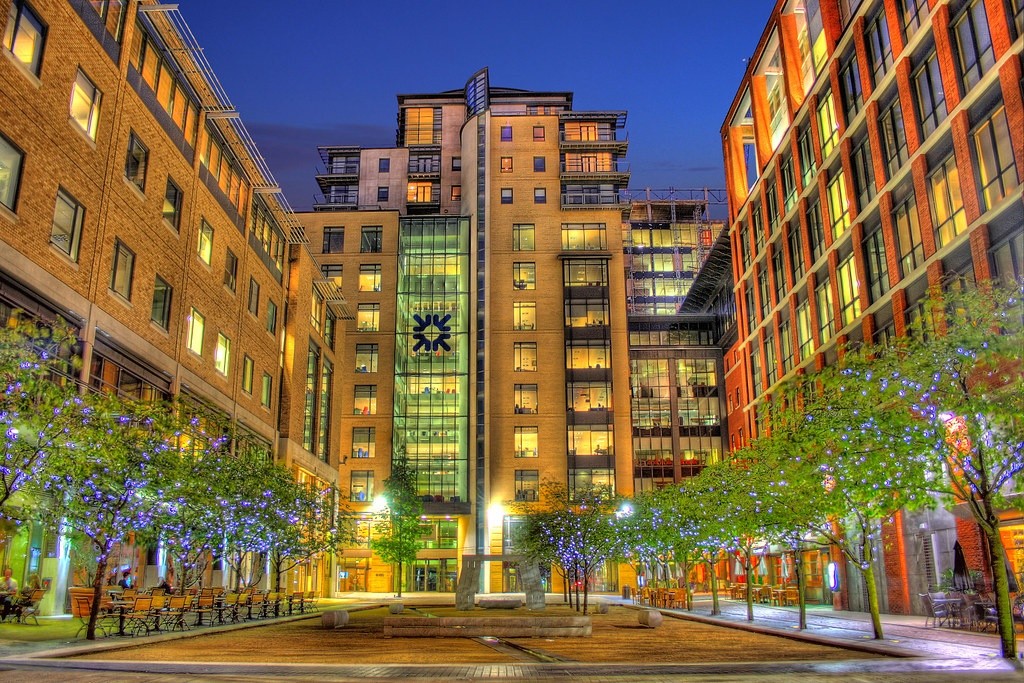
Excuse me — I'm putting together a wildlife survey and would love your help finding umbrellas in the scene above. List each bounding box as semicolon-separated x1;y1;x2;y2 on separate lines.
643;559;684;586
950;540;975;594
992;538;1019;594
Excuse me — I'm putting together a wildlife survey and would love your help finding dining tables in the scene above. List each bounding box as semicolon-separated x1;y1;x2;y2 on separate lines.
190;599;209;626
0;591;17;625
150;600;169;632
214;598;228;623
753;588;773;604
773;589;796;607
934;599;965;629
106;601;138;637
975;602;993;632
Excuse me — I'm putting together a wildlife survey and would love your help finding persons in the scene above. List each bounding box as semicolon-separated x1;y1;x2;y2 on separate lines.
119;572;134;590
158;577;171;595
16;574;40;623
0;568;18;623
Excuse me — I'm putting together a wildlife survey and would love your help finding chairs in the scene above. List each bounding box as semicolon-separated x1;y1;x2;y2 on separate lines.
67;584;321;639
917;591;1004;634
631;587;692;610
723;583;801;607
10;588;47;625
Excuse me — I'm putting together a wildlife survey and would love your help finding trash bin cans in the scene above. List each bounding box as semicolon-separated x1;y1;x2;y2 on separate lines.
622;584;631;600
